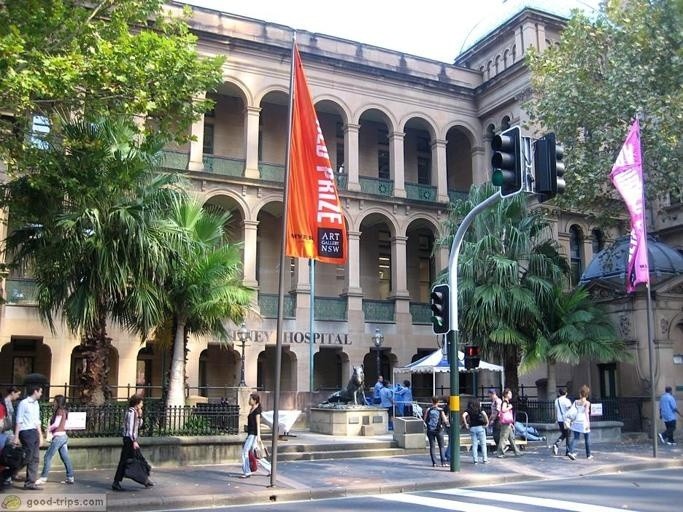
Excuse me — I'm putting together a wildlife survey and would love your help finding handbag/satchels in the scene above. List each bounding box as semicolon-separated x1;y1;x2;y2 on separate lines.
0;416;11;433
497;410;513;423
123;448;146;485
254;440;269;459
477;413;484;423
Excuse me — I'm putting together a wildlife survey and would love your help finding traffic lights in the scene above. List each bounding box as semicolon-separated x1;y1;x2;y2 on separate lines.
534;132;566;203
430;284;449;334
491;125;521;198
464;345;481;370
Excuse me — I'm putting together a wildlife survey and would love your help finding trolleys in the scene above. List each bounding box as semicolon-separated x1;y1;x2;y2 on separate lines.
478;410;528;450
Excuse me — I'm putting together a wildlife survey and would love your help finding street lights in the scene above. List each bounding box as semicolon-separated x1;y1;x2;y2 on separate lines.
372;327;385;376
236;323;251;387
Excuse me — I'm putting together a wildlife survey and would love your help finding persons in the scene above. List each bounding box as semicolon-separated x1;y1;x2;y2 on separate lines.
111;395;157;490
0;381;74;489
564;385;593;460
239;393;271;478
553;387;577;456
361;375;546;467
658;386;682;445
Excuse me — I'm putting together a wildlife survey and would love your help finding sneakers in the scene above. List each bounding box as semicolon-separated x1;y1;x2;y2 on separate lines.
666;440;677;444
442;463;450;467
433;463;442;467
587;454;593;459
658;433;665;444
566;452;575;459
553;444;558;455
59;480;73;484
35;480;46;485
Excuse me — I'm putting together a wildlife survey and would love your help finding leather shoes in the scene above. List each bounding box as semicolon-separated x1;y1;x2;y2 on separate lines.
23;484;42;490
144;481;155;487
111;482;121;490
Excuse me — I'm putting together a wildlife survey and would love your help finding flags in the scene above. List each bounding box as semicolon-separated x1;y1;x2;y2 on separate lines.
608;120;649;294
283;45;348;265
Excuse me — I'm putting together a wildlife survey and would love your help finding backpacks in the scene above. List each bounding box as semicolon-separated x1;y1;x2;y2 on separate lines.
425;407;441;432
